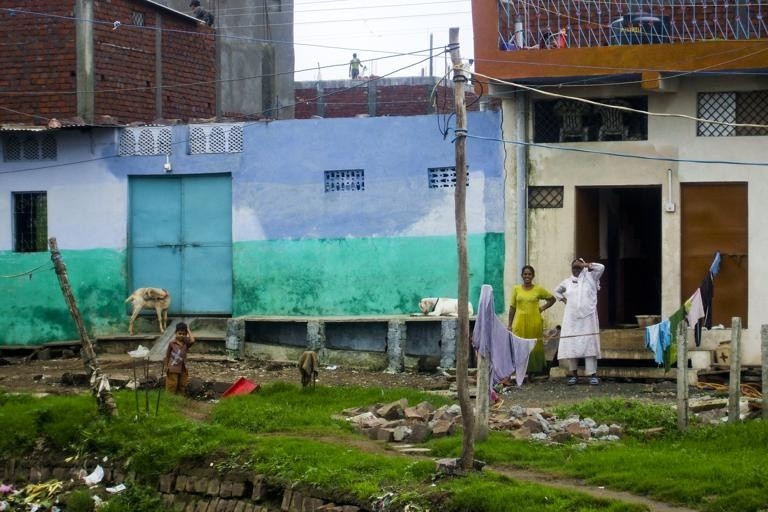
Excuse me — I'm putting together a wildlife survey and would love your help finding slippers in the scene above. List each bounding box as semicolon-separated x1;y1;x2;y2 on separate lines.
498;379;515;387
492;399;504;410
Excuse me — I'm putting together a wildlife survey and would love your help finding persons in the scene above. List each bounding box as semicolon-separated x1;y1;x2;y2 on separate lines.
348;52;363;79
189;1;216;28
550;257;607;387
505;262;554;385
161;320;196;399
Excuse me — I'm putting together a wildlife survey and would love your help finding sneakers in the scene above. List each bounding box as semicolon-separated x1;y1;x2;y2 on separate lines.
589;376;600;385
568;375;578;385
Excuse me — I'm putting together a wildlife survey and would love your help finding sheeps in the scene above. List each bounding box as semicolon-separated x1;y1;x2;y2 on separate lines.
412;298;474;320
124;287;172;335
